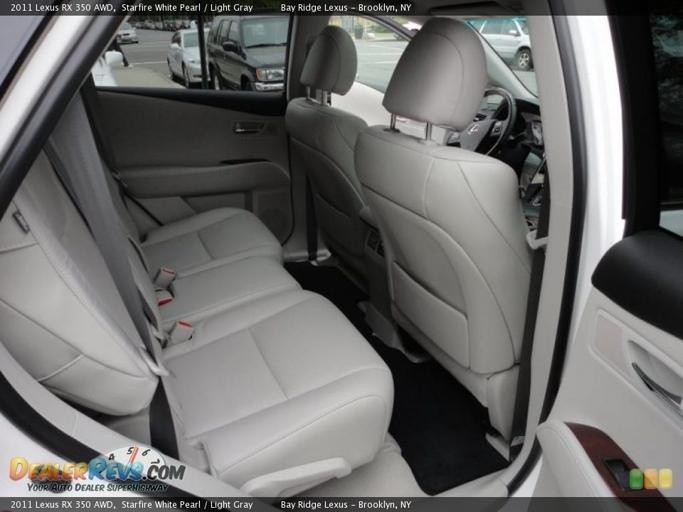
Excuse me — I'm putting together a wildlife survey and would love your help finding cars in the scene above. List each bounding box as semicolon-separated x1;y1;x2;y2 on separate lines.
166;28;212;87
0;1;680;510
131;19;208;31
395;21;422;39
116;23;140;44
463;18;534;72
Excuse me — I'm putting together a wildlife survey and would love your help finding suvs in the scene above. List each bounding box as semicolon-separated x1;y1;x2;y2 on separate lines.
207;15;292;92
